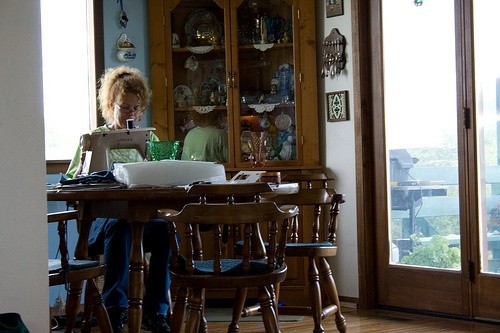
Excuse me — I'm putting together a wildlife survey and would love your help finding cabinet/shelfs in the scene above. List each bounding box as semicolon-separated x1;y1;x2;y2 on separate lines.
147;0;322;166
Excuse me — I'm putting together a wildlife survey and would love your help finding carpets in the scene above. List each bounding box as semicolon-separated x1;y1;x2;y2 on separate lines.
185;306;304;322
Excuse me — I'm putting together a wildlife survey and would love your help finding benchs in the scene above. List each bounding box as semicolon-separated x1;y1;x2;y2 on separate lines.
392;166;500;260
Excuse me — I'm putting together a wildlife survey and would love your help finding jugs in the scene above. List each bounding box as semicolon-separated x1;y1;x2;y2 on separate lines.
171;33;179;45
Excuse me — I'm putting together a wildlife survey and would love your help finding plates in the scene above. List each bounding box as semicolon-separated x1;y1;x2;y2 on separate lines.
274;114;292;129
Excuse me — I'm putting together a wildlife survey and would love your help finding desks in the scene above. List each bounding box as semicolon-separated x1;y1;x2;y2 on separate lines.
47;181;298;333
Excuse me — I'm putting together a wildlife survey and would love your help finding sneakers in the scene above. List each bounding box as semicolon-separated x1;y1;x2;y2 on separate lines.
104;301;128;333
140;315;171;333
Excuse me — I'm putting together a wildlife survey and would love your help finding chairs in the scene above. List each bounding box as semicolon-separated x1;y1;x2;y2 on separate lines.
47;171;347;333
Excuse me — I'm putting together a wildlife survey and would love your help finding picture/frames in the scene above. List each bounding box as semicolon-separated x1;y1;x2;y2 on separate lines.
325;90;350;122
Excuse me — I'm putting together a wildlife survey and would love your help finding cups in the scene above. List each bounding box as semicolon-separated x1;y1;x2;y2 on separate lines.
145;140;179;161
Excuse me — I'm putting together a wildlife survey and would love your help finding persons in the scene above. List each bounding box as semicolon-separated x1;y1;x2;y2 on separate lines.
180;79;230;163
64;66;181;333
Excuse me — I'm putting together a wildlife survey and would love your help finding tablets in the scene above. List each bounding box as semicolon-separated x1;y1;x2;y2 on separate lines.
83;130;150;179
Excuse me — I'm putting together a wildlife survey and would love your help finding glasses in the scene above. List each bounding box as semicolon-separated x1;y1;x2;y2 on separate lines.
115;103;144;117
